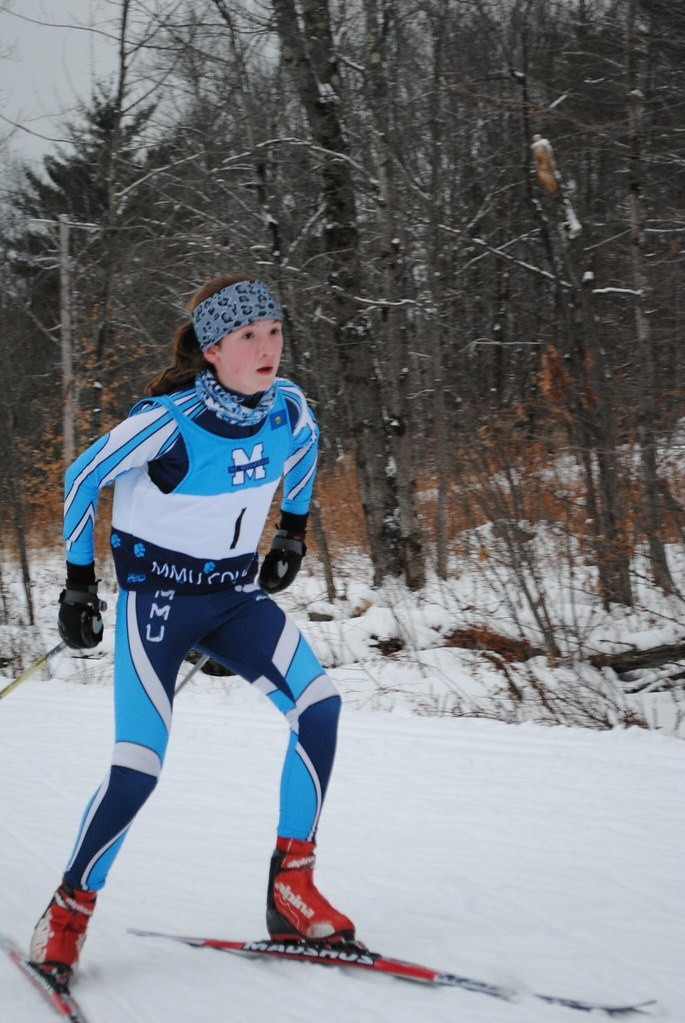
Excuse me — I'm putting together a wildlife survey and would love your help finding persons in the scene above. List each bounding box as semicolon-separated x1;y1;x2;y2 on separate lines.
27;269;359;993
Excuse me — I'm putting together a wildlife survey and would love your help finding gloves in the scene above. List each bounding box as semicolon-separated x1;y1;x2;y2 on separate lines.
57;578;107;650
256;524;308;594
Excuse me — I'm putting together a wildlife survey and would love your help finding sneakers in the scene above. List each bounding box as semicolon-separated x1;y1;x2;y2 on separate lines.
265;835;357;944
30;882;96;984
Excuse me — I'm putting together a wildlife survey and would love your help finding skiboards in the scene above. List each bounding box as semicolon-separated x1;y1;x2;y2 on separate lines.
0;927;660;1023
